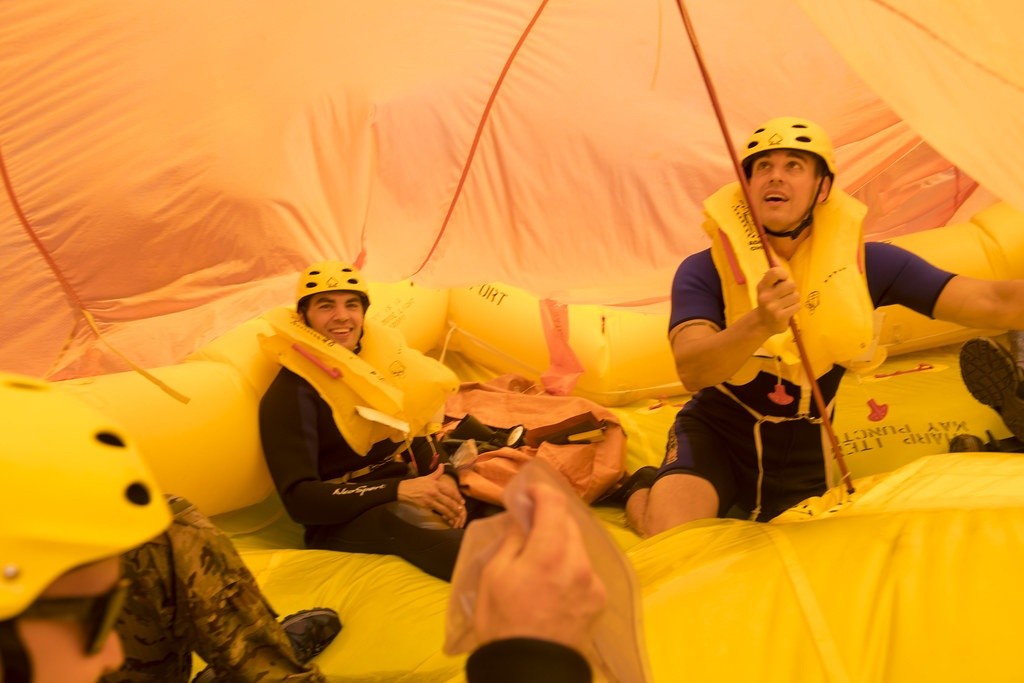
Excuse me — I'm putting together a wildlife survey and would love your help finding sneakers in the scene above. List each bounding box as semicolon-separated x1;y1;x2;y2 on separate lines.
192;607;343;683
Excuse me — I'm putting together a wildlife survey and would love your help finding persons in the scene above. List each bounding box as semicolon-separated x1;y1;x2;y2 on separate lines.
947;336;1023;455
259;260;497;587
598;116;1024;540
0;372;608;683
116;492;342;682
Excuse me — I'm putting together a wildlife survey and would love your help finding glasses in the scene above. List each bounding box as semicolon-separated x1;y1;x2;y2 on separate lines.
21;579;132;654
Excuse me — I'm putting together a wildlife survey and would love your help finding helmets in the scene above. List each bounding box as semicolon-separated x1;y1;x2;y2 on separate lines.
0;371;173;622
739;117;837;176
295;261;370;314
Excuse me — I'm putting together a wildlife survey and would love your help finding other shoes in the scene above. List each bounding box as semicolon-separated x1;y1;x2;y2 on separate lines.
958;334;1024;443
949;434;983;453
